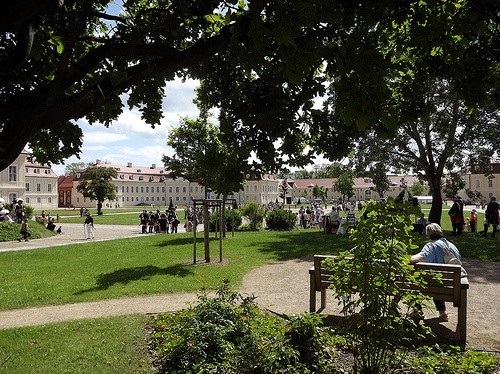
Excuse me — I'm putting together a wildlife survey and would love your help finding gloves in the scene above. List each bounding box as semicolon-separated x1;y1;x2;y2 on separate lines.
89;222;92;224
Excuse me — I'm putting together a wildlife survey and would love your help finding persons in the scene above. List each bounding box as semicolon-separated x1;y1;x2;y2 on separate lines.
385;195;388;204
444;196;500;240
110;202;120;208
409;223;462;322
360;296;402;316
411;213;431;235
139;200;363;235
0;198;95;242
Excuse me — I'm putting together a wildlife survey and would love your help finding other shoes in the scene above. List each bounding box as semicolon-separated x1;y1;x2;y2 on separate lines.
25;240;28;242
409;311;424;319
19;239;21;242
439;312;449;322
87;238;91;239
92;237;94;239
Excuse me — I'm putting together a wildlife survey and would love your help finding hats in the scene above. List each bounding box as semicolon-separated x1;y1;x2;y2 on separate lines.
157;210;160;213
452;196;462;200
149;211;153;213
166;210;169;212
171;208;175;210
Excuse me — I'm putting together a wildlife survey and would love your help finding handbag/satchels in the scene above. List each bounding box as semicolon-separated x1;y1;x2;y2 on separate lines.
451;216;460;223
20;231;25;235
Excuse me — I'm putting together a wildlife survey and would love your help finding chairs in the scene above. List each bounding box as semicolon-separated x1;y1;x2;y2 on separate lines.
157;219;167;234
347;214;356;237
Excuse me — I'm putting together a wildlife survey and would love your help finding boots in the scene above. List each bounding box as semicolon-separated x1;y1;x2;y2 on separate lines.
167;227;170;234
149;228;153;233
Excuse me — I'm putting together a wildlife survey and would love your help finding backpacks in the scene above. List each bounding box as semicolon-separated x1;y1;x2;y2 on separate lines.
434;241;467;277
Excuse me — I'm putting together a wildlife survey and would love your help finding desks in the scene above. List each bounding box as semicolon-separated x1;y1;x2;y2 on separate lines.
411;221;431;235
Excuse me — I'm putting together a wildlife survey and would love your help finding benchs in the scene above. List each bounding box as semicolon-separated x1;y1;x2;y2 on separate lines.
308;254;469;352
321;215;340;233
